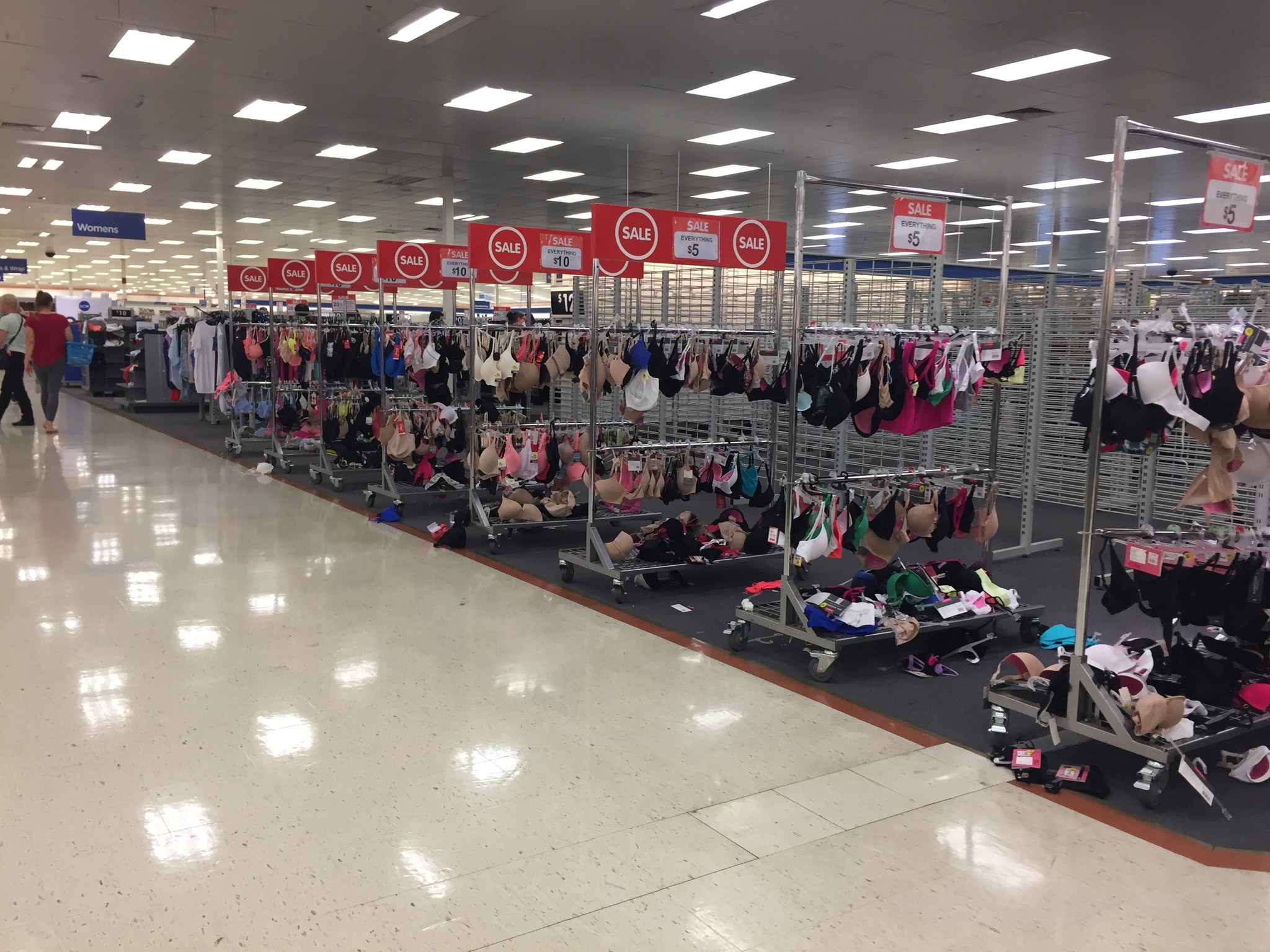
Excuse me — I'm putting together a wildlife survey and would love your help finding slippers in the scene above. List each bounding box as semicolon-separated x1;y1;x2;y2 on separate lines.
42;422;59;435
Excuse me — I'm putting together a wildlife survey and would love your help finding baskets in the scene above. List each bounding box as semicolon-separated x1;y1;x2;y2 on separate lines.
65;335;97;368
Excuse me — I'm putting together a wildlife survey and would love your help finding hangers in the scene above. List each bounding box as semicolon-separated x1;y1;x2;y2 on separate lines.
251;315;1267;568
161;306;249;328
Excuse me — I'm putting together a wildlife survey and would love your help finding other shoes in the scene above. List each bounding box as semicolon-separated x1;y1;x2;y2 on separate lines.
35;378;42;394
9;393;19;404
12;419;35;427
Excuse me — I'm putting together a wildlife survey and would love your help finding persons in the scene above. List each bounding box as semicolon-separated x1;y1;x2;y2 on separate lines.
23;290;73;434
0;293;35;426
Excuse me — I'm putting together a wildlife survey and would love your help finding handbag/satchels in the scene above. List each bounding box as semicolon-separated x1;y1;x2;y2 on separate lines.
0;347;9;370
